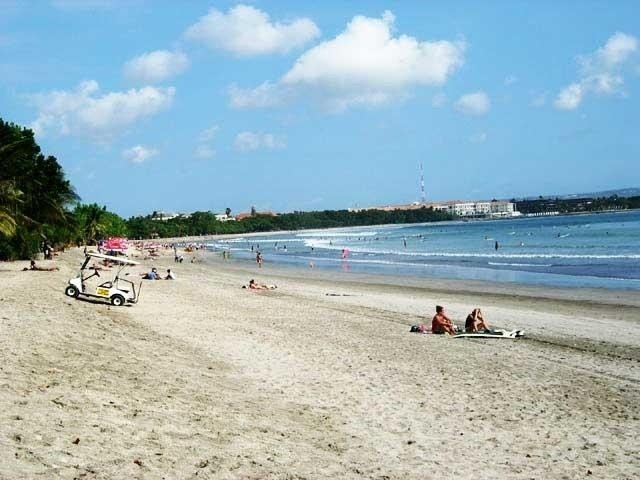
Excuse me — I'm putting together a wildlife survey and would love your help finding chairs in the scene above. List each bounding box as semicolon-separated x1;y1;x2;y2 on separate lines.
100;277;118;288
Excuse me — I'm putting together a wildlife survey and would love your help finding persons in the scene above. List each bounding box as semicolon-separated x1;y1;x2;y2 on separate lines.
96;238;287;290
29;261;60;272
432;306;457;335
84;247;87;257
465;308;492;334
43;240;54;259
342;249;346;259
